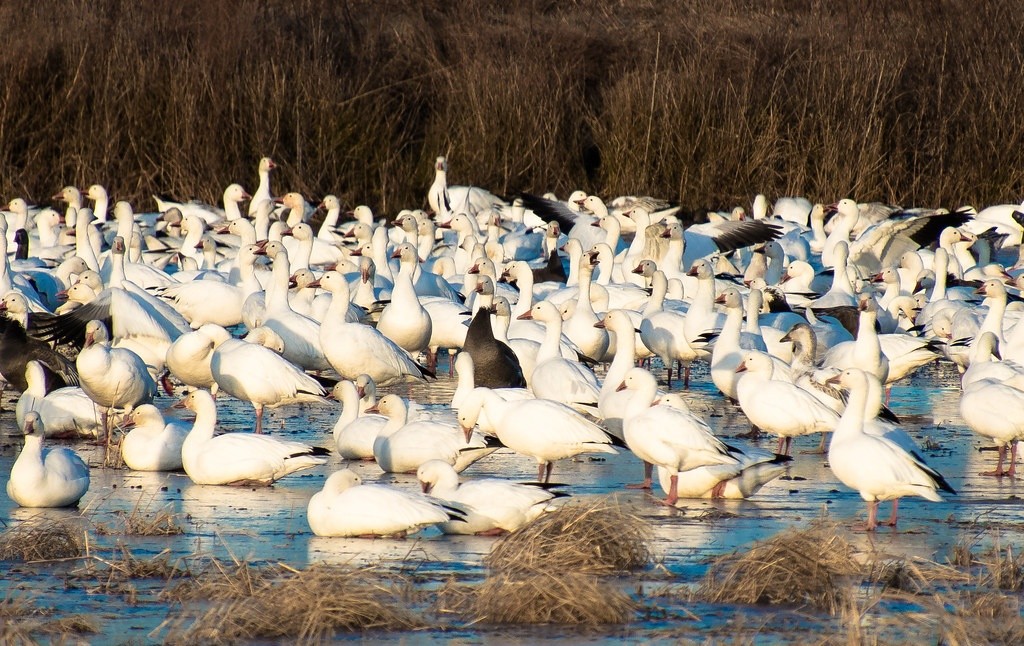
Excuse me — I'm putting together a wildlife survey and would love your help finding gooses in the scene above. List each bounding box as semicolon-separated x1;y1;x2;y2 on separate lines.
0;155;1024;538
